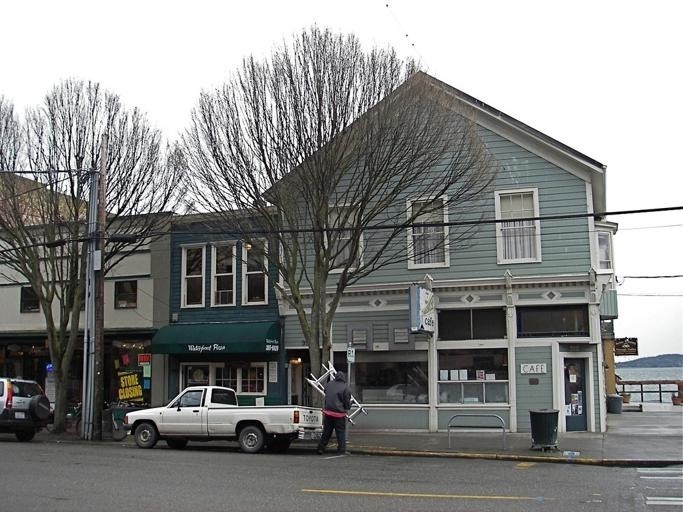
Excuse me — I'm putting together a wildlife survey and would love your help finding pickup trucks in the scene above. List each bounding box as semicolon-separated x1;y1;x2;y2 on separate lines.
121;385;325;454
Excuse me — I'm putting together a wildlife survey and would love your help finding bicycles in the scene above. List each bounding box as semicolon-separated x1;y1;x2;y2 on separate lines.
71;399;130;441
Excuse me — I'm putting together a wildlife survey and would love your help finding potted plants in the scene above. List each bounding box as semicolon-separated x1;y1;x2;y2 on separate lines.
670;392;682;406
620;394;631;404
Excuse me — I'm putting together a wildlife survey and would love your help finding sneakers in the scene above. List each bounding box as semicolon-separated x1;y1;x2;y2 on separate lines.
317;448;352;456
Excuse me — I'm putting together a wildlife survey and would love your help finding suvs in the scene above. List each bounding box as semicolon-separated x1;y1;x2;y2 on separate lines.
0;377;51;442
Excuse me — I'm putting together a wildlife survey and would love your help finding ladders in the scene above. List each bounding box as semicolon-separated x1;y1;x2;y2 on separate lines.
304;360;368;426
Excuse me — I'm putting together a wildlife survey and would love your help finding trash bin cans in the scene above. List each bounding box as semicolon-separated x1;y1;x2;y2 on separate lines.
606;394;623;414
528;408;560;452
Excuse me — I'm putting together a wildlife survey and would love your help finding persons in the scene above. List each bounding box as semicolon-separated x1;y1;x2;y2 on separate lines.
315;371;351;455
